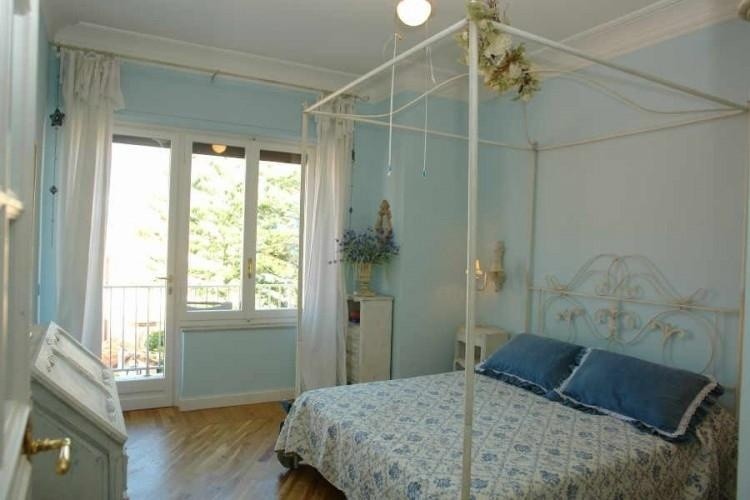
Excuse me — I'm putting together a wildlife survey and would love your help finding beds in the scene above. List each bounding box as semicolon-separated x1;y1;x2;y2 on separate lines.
275;15;749;500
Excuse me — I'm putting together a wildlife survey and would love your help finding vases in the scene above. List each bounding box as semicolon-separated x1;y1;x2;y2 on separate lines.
352;263;375;297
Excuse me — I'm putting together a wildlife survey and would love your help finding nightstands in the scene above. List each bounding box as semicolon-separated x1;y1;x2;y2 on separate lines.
454;326;509;370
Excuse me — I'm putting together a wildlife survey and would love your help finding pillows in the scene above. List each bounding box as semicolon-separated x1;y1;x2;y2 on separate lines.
475;333;585;395
550;346;726;448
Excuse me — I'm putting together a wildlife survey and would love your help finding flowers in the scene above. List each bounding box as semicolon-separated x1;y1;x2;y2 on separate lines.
326;229;401;265
463;0;540;101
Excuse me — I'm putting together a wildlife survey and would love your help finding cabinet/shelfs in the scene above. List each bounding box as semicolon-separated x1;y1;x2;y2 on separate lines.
345;293;394;384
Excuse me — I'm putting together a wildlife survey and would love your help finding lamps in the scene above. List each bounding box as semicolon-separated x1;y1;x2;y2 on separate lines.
212;145;227;154
465;241;506;293
396;0;432;27
